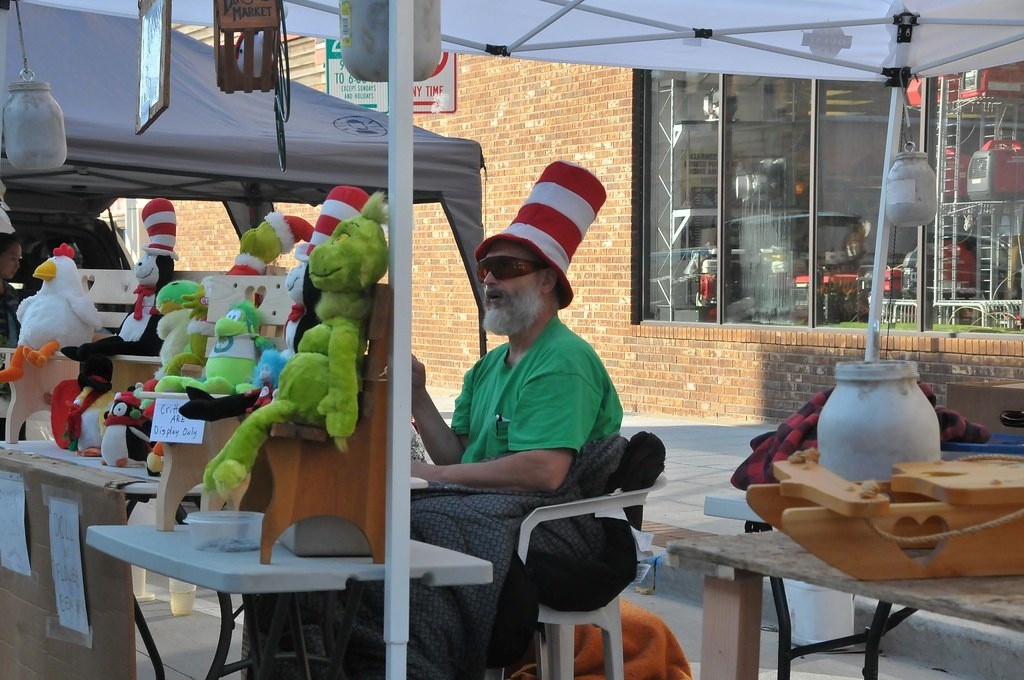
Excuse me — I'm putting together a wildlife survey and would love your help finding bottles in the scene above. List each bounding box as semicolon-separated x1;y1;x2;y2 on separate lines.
816;359;940;484
4;81;67;170
886;152;937;226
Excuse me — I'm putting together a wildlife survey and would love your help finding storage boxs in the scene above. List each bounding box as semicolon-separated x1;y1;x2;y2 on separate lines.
943;379;1024;435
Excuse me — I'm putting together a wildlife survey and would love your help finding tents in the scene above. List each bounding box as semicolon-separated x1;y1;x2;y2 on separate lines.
20;1;1024;363
0;2;486;362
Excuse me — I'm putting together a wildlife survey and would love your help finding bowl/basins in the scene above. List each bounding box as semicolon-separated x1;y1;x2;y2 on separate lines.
183;512;264;552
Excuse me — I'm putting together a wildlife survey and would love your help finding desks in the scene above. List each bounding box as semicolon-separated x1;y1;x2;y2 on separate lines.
0;441;428;680
85;525;493;680
704;488;922;680
667;530;1023;679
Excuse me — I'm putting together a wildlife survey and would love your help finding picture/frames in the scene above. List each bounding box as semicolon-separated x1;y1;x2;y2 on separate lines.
135;0;170;136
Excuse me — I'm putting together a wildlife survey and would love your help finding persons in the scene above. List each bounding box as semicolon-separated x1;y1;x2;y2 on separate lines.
1;232;22;396
408;160;628;678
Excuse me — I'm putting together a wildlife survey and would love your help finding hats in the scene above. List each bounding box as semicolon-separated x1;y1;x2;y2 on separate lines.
474;160;606;309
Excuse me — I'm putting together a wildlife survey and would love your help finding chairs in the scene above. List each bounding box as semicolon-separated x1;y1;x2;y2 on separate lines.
484;467;670;679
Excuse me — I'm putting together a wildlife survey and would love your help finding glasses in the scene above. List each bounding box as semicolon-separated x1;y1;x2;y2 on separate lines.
476;256;547;283
0;253;23;262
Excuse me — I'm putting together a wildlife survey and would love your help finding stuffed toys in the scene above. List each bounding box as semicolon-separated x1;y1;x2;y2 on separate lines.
1;185;390;507
